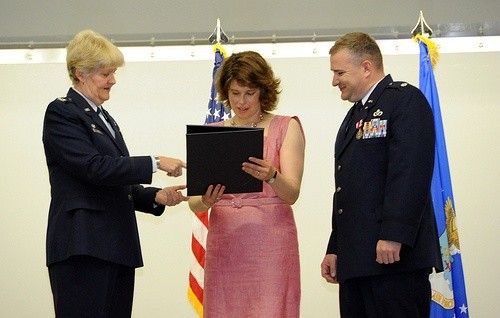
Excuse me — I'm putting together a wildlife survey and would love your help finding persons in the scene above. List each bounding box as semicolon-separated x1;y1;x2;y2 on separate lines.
42;28;187;318
321;33;444;318
186;50;305;318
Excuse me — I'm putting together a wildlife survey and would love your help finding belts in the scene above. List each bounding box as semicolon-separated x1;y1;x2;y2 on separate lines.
213;197;286;207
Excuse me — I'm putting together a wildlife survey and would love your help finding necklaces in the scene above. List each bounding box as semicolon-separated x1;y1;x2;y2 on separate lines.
230;110;264;128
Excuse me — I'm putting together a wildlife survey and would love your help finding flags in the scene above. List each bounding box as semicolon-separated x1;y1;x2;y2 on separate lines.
185;42;233;318
411;33;469;318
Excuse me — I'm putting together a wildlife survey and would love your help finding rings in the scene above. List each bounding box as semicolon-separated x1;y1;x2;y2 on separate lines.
216;197;221;200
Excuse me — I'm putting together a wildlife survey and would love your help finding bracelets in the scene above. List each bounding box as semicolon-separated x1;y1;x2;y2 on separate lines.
201;195;215;209
153;189;161;205
154;155;161;170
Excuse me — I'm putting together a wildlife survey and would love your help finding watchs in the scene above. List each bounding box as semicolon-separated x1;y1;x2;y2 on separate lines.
265;168;277;185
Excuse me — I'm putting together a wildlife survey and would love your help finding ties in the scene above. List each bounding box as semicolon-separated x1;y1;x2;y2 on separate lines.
344;100;363;139
